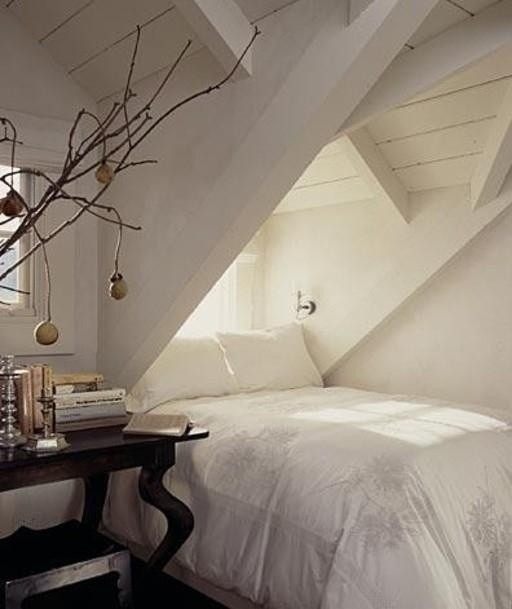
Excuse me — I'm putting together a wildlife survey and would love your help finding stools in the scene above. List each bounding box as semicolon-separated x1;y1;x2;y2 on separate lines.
0;519;134;608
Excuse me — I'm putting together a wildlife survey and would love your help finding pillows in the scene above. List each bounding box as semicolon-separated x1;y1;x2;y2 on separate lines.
124;323;323;413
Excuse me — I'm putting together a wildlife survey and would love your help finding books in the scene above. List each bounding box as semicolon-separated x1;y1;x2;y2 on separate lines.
0;361;129;435
122;413;196;438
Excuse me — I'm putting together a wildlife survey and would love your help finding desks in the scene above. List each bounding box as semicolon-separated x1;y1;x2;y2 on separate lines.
0;410;210;609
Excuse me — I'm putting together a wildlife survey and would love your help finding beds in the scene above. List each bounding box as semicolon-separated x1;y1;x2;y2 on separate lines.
102;385;512;609
